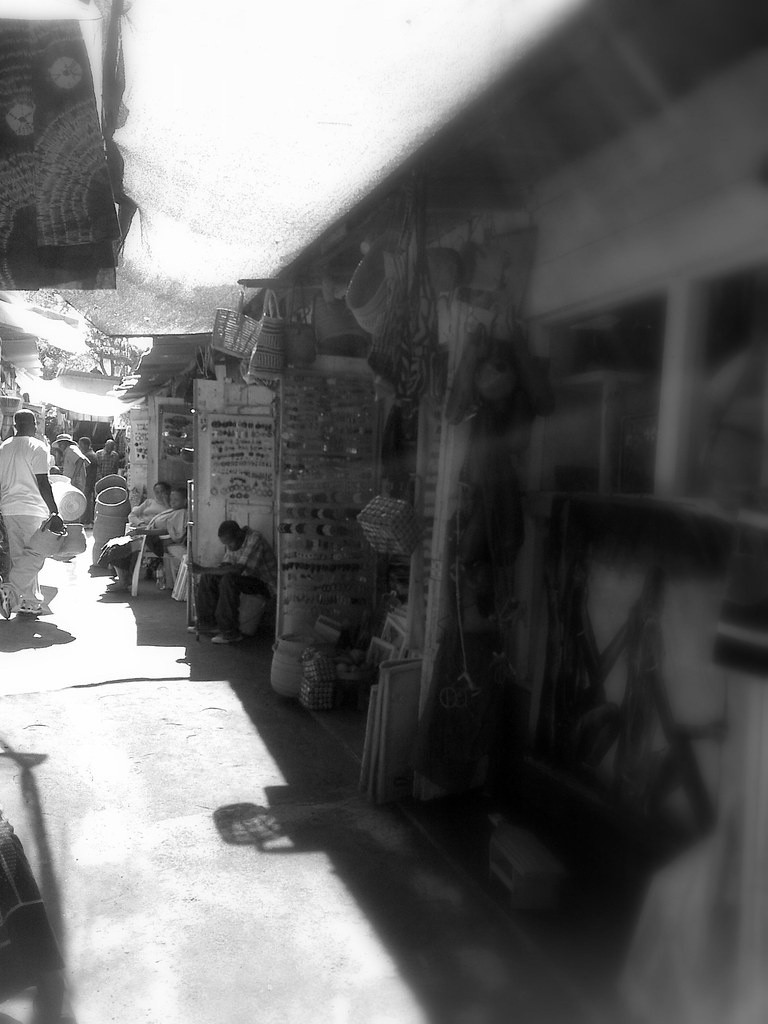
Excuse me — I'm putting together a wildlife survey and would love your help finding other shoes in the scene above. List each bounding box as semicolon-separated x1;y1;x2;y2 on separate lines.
211;631;244;643
0;587;12;618
17;602;42;614
106;577;132;591
187;625;218;633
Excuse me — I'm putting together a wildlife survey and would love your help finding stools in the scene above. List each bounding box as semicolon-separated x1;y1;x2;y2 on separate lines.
488;820;568;912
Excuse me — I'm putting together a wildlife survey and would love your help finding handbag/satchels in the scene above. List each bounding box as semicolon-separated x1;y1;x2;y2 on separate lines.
29;514;67;556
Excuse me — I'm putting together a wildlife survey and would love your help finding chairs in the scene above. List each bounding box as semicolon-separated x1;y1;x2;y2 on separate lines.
131;534;172;597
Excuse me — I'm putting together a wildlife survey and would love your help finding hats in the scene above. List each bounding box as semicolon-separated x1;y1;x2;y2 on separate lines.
52;433;77;448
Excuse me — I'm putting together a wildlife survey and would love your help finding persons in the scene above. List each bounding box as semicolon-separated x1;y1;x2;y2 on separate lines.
0;409;192;619
188;519;276;645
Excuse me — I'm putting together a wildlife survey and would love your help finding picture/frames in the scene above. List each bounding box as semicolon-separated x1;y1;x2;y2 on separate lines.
365;607;411;668
